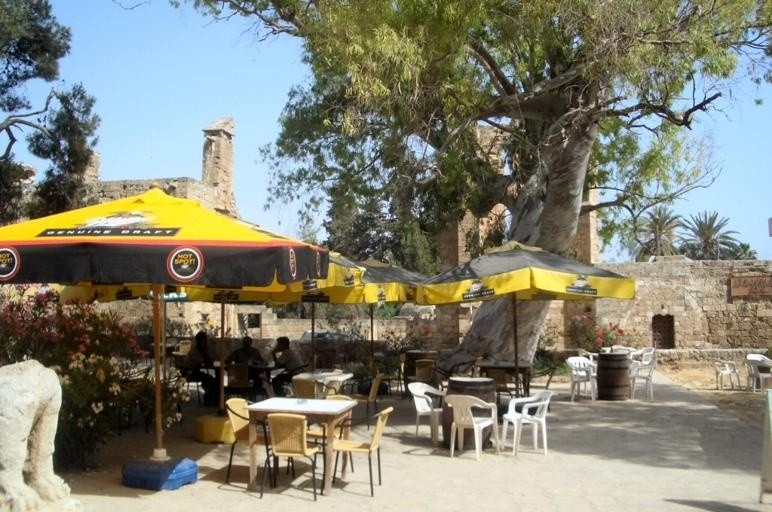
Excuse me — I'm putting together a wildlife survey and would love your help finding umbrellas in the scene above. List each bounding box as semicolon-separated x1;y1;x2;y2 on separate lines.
270;247;432;378
44;239;369;413
1;187;331;447
410;238;636;397
57;281;291;382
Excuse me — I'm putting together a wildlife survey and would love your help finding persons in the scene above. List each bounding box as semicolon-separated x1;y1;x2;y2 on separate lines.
224;335;265;403
271;336;306;397
180;330;218;408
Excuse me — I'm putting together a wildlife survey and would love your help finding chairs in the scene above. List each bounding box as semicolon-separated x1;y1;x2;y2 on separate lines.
711;357;742;391
746;353;772;393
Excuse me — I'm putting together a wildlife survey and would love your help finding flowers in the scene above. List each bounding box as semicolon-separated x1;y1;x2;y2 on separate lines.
578;316;626;351
404;316;434;351
